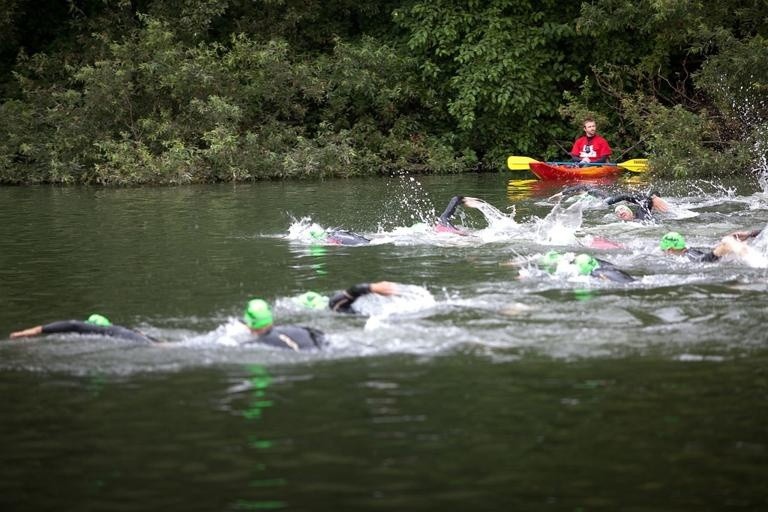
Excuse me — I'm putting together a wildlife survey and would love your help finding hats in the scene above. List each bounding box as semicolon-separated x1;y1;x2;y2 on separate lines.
244;294;272;329
660;233;688;252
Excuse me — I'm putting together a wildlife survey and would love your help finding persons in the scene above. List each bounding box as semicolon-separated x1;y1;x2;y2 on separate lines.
7;313;161;347
299;282;402;322
732;224;768;246
431;192;467;241
656;231;727;262
543;248;642;295
604;188;667;224
305;219;371;246
549;183;610;211
570;116;611;166
230;295;328;357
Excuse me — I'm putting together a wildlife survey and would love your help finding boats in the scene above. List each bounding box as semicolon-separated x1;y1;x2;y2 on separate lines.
530;159;623;183
526;180;624;192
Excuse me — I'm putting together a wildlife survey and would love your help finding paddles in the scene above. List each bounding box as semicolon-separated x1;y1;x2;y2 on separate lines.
507;155;648;173
